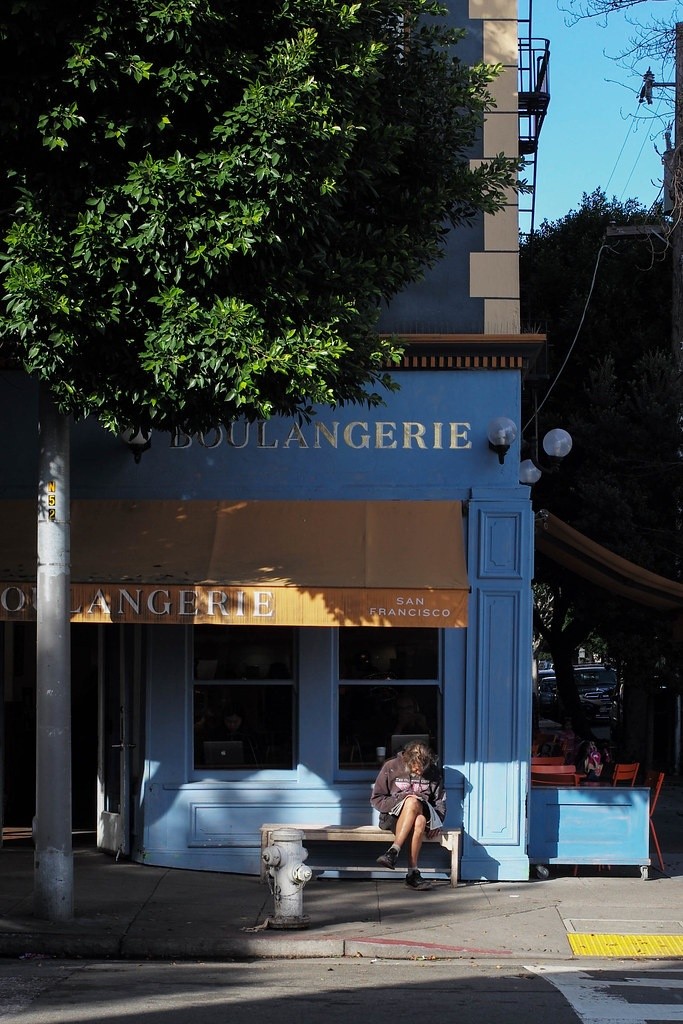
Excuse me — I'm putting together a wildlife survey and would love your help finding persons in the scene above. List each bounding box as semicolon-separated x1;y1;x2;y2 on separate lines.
375;694;429;747
370;740;446;890
207;703;257;763
557;715;580;737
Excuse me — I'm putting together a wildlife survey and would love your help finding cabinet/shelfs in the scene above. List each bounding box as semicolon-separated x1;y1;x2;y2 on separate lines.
531;785;652;881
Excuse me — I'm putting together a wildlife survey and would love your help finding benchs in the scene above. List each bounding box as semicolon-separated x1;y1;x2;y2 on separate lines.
260;822;463;889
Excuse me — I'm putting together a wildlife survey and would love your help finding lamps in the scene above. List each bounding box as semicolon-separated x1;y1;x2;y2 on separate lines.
519;428;574;495
486;415;518;466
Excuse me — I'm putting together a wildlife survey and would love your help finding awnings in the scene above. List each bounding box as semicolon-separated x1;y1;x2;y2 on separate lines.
535;511;683;609
0;500;471;628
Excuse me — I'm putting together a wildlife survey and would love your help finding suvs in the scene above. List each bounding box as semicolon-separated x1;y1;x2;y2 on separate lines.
537;663;624;725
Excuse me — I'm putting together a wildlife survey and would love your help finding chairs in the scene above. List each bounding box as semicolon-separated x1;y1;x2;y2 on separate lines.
531;728;664;878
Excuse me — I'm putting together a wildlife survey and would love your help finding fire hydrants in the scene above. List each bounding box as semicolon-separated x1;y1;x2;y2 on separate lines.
261;826;312;932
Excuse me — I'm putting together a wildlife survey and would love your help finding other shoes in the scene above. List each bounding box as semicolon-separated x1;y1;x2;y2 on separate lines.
378;847;399;870
404;869;433;891
